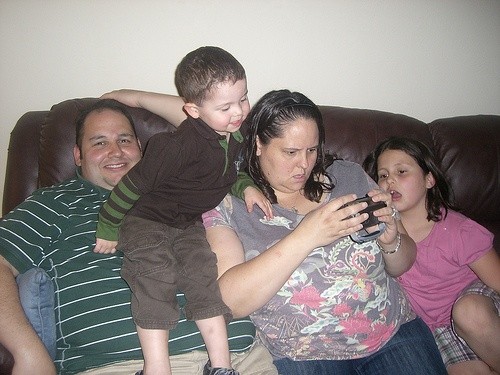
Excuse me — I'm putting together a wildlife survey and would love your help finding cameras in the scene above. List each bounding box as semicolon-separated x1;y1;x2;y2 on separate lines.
340;197;387;231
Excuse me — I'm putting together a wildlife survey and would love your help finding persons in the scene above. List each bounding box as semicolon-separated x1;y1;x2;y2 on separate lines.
201;89;448;375
0;89;278;375
93;46;274;375
362;136;500;375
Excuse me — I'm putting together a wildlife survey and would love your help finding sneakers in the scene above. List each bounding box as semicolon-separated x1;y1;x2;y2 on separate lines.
203;359;240;375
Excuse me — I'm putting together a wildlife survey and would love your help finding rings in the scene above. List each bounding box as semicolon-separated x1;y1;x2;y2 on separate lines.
392;208;396;217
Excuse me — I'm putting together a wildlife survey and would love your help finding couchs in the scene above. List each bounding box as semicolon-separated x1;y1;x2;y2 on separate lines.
0;98;500;375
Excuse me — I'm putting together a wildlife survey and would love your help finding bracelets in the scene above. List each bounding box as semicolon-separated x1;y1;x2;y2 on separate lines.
376;232;401;254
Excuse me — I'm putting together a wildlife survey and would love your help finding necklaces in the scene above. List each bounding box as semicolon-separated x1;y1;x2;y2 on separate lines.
291;192;299;213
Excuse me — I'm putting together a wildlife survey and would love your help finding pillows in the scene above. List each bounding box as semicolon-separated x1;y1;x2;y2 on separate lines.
15;268;55;364
